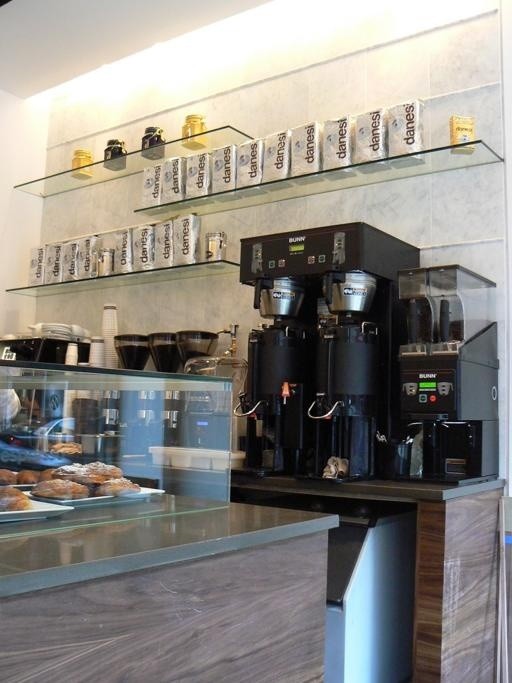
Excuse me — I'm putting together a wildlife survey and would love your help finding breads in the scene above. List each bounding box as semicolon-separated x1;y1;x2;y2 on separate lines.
0;442;142;512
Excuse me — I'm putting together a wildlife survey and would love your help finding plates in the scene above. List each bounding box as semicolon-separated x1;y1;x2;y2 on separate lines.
0;468;166;525
41;322;73;342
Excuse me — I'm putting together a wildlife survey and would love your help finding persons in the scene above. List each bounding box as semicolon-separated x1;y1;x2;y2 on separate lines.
1;388;44;432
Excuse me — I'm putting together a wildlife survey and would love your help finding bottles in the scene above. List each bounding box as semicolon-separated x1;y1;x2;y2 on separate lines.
69;111;208;181
64;342;80;366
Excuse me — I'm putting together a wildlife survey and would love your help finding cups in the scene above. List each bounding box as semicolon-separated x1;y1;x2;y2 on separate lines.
96;247;115;278
204;232;228;269
87;302;121;370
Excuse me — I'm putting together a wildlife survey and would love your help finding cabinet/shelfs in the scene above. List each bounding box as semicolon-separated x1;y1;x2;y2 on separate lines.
5;125;503;298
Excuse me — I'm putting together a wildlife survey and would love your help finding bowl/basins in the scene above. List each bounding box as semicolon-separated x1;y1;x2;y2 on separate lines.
113;329;220;373
